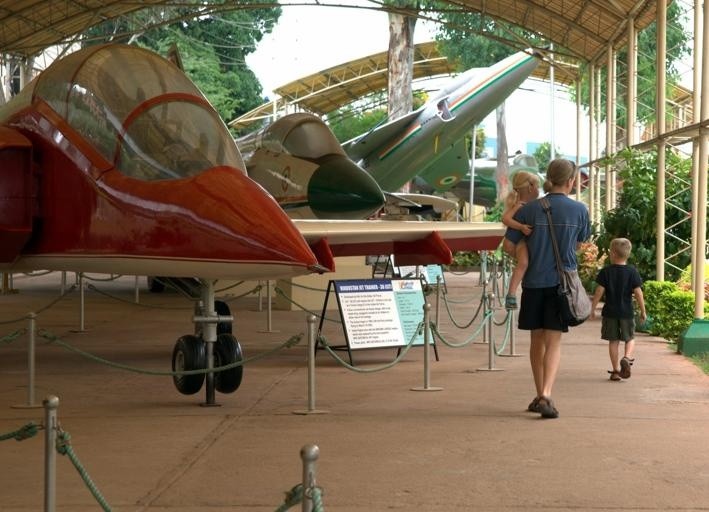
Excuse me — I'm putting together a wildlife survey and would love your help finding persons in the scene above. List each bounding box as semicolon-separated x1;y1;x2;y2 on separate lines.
503;158;591;418
589;237;647;380
503;171;540;309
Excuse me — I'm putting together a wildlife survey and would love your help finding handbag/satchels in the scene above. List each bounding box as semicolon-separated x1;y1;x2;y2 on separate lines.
559;270;592;327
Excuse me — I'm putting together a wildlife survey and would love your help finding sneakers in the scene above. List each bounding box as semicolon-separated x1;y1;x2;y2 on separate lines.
620;357;634;378
528;397;557;418
608;371;621;379
505;296;516;310
